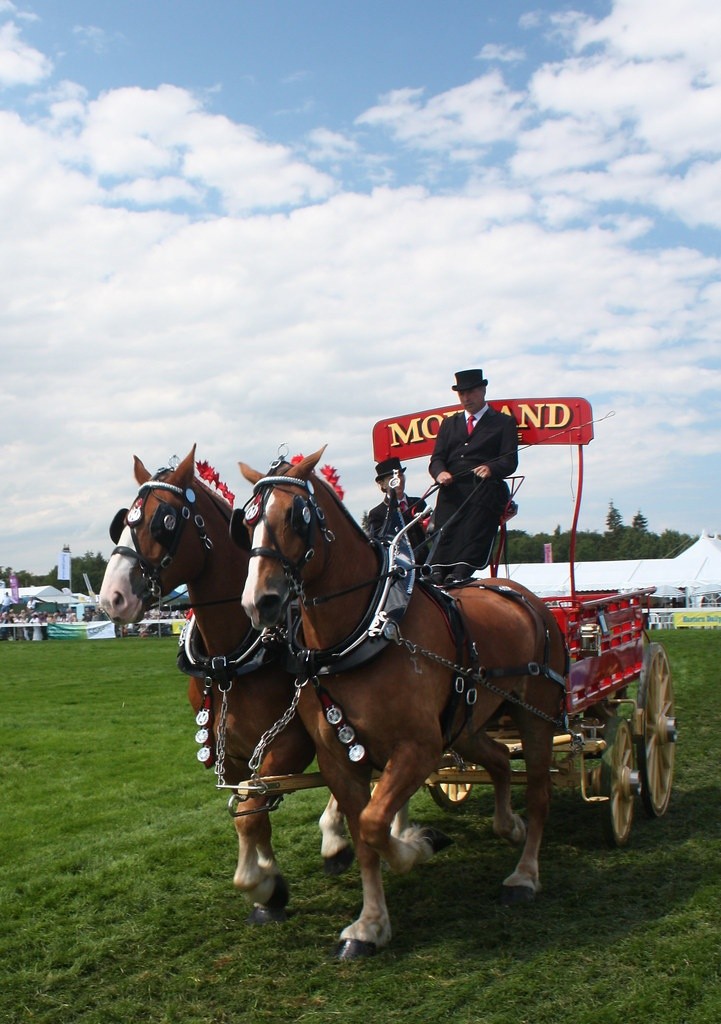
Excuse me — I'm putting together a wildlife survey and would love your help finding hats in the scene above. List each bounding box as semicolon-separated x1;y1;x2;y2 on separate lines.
375;456;406;482
451;369;488;391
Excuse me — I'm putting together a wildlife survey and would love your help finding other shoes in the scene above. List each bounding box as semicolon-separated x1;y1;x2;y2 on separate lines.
423;572;461;585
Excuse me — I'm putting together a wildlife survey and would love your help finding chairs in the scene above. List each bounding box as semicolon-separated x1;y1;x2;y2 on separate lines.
410;474;525;581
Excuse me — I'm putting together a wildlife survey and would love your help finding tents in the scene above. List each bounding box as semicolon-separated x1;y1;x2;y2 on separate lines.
471;531;721;593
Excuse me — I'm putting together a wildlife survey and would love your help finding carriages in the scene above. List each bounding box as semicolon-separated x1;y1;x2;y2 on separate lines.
100;393;687;960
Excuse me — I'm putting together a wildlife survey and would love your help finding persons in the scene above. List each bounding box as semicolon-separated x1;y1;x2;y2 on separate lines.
364;456;428;569
0;587;195;642
421;370;520;583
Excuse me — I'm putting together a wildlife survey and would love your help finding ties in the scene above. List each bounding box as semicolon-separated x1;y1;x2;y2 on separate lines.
466;416;476;437
400;501;408;516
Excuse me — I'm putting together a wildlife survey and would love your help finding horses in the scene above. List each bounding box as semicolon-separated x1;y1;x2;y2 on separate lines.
96;443;412;921
241;445;570;967
